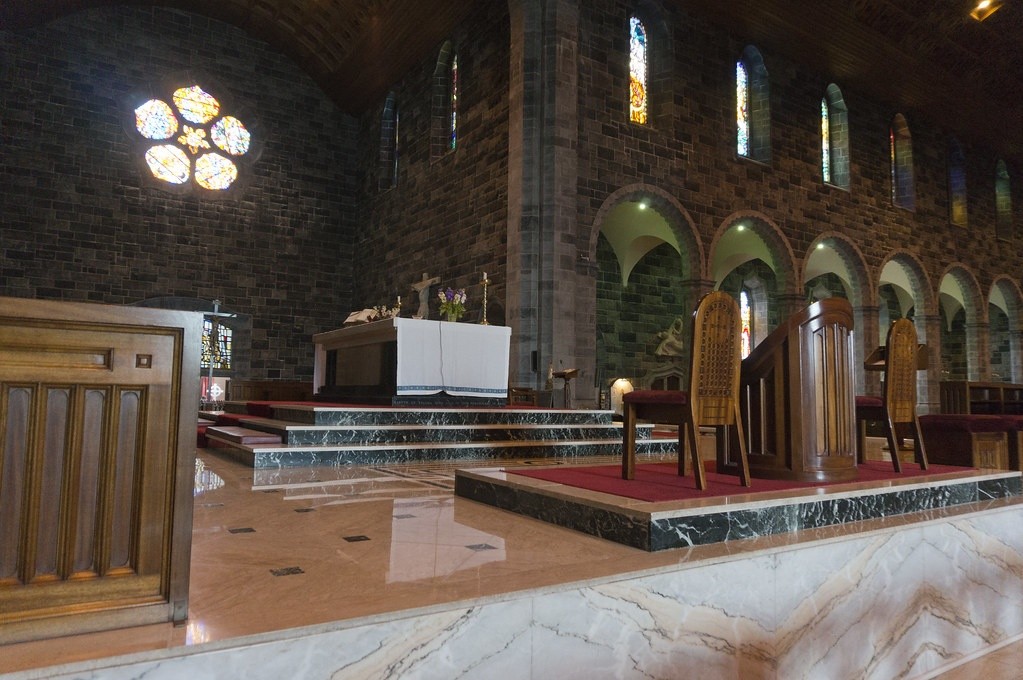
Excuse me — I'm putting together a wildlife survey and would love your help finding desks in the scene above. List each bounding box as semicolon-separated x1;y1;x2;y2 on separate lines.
313;317;512;407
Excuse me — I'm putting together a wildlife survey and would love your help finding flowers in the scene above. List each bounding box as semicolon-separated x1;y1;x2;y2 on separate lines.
438;287;467;321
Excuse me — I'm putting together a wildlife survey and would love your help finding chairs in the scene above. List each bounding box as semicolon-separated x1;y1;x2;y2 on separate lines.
620;291;753;491
855;318;929;475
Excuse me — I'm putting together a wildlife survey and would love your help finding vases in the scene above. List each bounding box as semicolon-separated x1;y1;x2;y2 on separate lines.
447;311;458;323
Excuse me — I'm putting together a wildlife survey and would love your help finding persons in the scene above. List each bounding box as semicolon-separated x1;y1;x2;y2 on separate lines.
414;280;436;319
